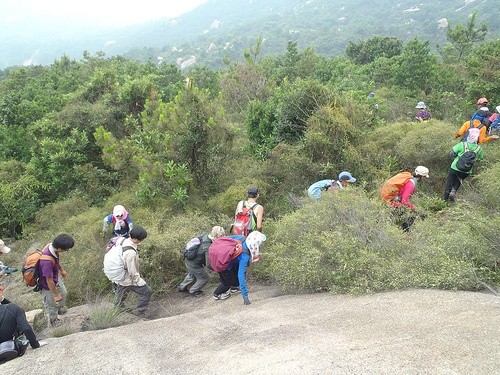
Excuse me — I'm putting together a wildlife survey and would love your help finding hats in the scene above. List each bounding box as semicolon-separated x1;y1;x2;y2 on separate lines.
339;172;356;183
246;231;266;263
496;106;500;114
0;239;11;254
415;166;430;179
208;226;226;242
467;128;480;144
415;102;427;110
248;187;258;194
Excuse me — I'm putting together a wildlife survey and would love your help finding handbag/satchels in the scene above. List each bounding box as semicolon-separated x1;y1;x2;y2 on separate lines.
0;329;30;365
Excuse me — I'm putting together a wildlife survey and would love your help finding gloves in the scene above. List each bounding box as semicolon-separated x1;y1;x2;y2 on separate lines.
244;300;250;305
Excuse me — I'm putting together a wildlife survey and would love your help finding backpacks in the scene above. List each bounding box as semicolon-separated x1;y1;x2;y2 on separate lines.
308;180;341;202
380;172;417;207
477;111;492;128
457;142;481;172
208;236;250;272
461;121;483;144
22;243;56;292
230;200;258;236
183;234;212;261
103;236;140;281
491;114;500;139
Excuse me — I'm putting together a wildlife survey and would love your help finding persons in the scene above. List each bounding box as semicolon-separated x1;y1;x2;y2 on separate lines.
0;285;46;365
415;102;430;123
213;231;266;305
368;93;378;121
0;239;18;279
39;233;74;328
102;205;133;294
390;166;430;233
456;98;500;145
443;128;484;207
235;186;264;233
328;171;356;190
176;226;225;298
103;227;152;319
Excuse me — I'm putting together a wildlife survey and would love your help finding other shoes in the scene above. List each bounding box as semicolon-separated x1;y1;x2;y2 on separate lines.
178;286;204;297
52;308;67;327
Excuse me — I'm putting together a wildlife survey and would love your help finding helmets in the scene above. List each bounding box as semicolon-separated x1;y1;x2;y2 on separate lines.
476;98;488;104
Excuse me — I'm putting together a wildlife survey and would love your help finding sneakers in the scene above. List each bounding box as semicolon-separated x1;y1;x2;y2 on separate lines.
213;287;242;300
